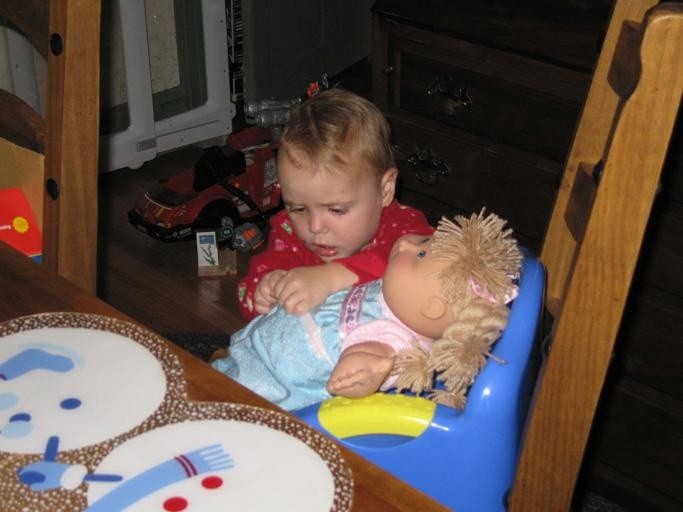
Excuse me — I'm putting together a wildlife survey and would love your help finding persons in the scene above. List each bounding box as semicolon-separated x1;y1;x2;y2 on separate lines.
197;208;523;409
237;89;436;323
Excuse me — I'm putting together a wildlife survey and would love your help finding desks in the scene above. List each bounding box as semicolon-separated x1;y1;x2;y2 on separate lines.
0;237;455;512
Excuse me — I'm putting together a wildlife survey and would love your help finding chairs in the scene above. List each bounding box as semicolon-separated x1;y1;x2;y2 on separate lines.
0;0;99;295
